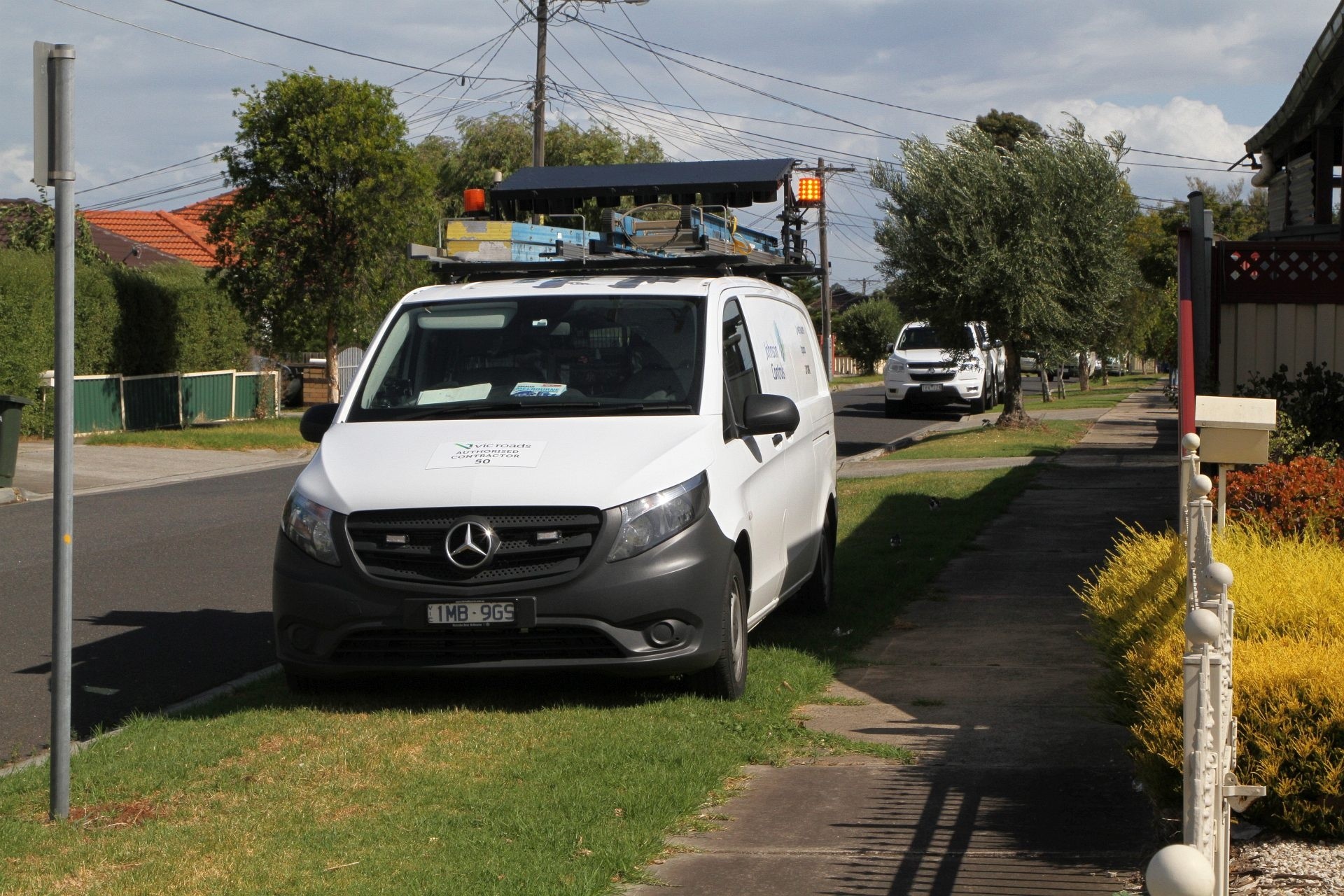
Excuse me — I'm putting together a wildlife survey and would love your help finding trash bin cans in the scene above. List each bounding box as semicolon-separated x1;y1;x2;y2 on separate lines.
1;394;33;489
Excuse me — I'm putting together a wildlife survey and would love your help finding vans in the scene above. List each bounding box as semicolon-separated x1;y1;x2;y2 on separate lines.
269;275;836;702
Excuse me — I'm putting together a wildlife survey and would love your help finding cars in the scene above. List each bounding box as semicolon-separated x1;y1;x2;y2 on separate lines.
1019;348;1121;382
883;319;1007;416
243;355;302;407
